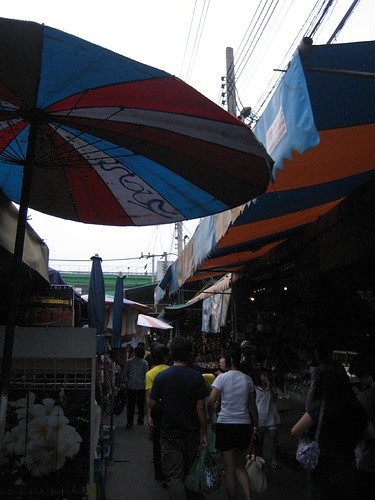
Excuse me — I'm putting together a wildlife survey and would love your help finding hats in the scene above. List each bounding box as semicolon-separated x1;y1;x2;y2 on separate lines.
240;340;254;349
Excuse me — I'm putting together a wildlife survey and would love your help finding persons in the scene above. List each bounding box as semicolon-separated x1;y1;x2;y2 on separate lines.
207;344;259;500
147;336;210;500
104;331;283;489
290;359;367;500
348;349;375;500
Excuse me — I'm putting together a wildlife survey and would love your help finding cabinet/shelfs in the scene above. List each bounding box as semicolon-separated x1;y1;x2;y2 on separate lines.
0;325;104;500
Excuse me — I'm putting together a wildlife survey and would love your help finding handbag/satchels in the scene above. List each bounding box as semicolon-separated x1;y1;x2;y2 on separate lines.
185;447;207;494
296;400;325;470
200;447;220;490
244;432;267;492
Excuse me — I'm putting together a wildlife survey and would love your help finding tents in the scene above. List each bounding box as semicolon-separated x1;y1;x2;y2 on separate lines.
79;294;153;347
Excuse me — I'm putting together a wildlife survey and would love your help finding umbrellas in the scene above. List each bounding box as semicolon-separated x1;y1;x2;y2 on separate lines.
0;17;276;450
113;272;126;365
88;253;107;405
136;313;174;330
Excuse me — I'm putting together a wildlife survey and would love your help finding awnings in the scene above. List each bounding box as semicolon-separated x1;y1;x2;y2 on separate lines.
157;304;203;324
184;272;232;306
153;38;375;306
124;280;160;304
0;192;86;317
